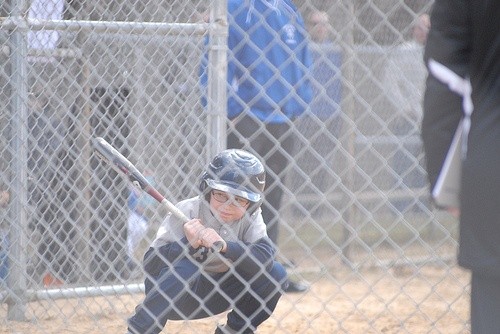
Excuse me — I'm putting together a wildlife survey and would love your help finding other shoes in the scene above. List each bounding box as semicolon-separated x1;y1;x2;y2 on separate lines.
282;278;306;293
214;325;226;334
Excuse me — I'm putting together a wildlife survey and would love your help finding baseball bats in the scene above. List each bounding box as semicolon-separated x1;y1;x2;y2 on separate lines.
93;138;224;254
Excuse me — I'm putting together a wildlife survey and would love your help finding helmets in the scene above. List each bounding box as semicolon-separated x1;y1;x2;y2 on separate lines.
199;147;265;203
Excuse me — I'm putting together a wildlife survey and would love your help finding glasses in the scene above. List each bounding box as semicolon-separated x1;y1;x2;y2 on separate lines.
208;188;249;208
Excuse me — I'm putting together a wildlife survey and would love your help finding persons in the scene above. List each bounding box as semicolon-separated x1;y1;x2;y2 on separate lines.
290;4;344;218
423;1;500;334
197;0;314;296
126;146;286;334
380;14;430;217
